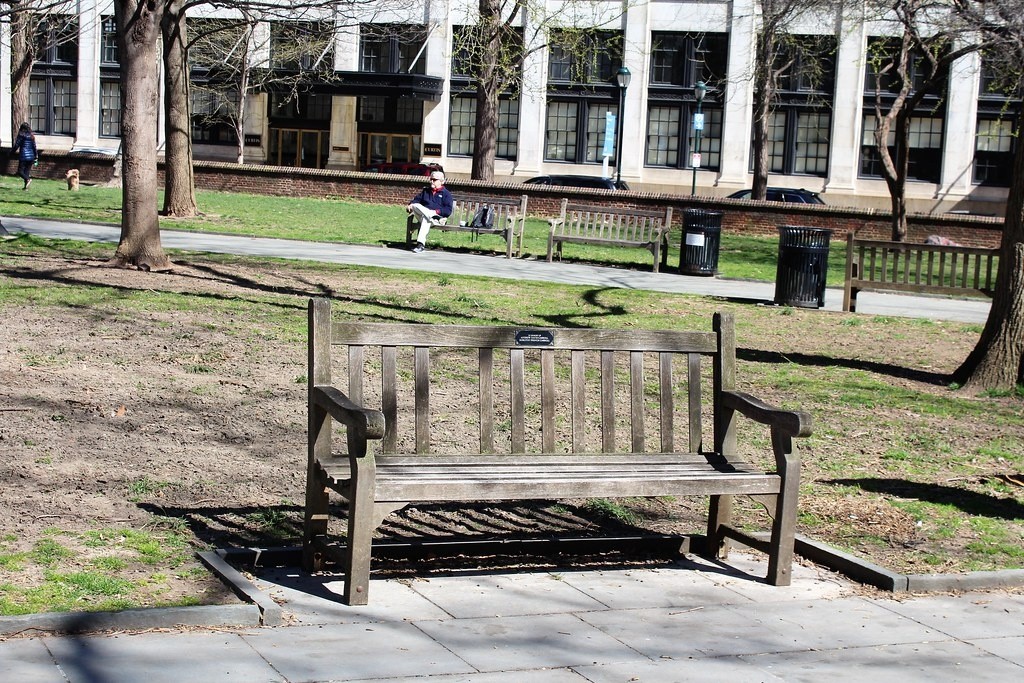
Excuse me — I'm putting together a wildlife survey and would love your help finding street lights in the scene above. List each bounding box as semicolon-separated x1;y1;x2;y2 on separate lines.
688;81;708;196
616;66;631;191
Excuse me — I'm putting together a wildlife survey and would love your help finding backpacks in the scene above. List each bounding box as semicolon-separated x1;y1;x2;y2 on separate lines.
470;205;494;228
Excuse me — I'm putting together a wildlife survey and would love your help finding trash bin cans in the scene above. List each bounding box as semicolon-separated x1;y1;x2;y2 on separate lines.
773;225;836;309
677;209;725;277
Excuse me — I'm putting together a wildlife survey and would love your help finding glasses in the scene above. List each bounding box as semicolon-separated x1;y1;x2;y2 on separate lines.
430;178;442;181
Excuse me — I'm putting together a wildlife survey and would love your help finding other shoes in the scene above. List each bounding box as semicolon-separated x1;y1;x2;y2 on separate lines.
25;179;32;188
439;217;448;226
22;188;28;191
413;243;425;253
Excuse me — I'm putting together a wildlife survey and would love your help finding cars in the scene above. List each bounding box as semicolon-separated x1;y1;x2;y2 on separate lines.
522;175;630;190
360;162;444;177
725;185;826;204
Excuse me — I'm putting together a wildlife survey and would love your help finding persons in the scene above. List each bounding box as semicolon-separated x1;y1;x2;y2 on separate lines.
406;171;453;253
10;122;38;191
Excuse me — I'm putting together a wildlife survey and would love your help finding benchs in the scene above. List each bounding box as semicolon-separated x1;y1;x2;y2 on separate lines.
406;195;529;259
843;232;1001;312
548;199;674;273
304;299;814;606
